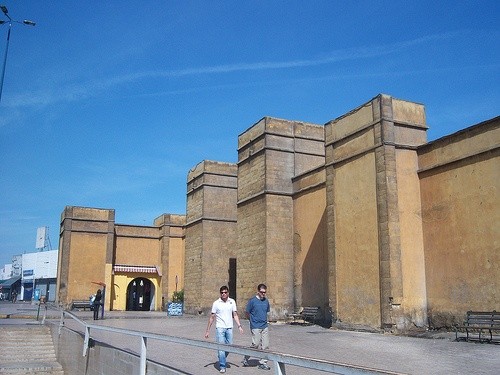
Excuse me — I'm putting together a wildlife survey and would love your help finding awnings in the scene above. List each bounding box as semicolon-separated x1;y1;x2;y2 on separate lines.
0;277;22;288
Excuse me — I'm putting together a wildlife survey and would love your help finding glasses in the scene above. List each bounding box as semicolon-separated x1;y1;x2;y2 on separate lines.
259;291;266;293
221;292;228;294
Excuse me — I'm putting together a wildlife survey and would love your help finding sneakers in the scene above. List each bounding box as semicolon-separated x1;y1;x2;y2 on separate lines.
220;368;226;373
243;359;250;367
257;363;271;371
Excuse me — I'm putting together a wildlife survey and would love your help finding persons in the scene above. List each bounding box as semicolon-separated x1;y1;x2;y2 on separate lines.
205;286;244;372
93;289;102;320
241;284;271;370
11;290;17;302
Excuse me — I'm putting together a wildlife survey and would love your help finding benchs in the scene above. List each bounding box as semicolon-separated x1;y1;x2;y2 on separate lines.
450;310;500;345
70;299;91;311
285;307;320;327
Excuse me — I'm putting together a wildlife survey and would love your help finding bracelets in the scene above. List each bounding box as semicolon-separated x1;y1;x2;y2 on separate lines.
238;325;242;328
